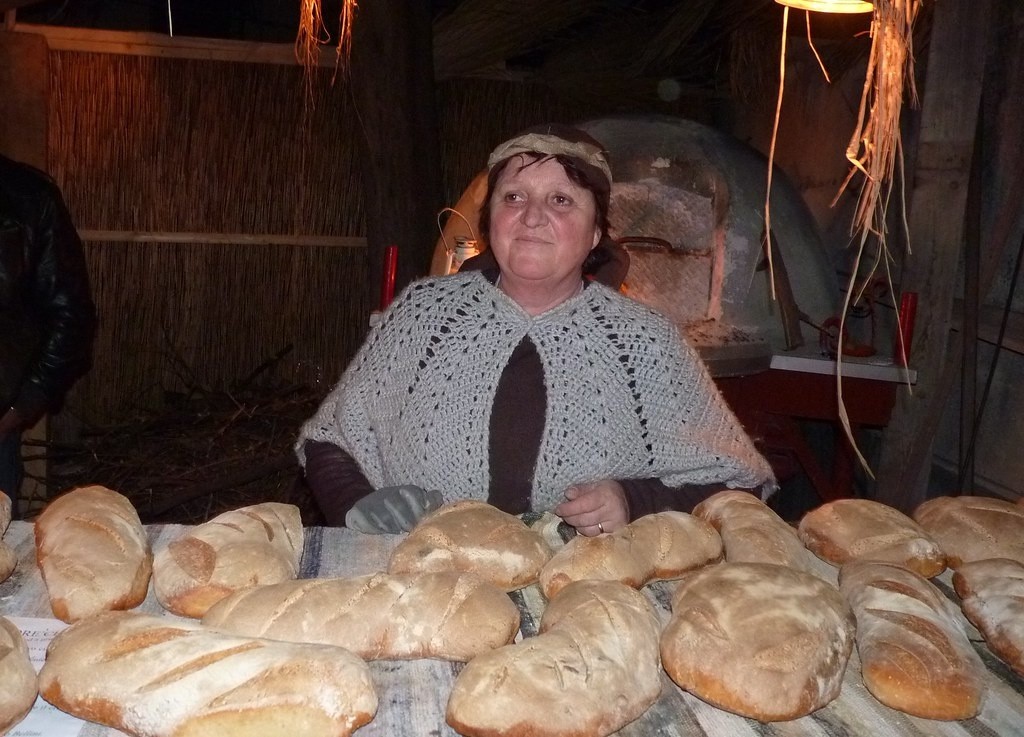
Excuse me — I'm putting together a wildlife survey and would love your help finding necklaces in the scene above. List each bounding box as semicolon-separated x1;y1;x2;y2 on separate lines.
494;272;584;321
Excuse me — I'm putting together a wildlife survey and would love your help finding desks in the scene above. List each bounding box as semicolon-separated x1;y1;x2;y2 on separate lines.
0;521;1024;737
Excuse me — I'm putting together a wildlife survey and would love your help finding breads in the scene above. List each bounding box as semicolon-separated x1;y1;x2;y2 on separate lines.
0;485;1024;737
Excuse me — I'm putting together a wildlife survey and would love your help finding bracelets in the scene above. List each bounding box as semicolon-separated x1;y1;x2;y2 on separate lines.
11;406;26;426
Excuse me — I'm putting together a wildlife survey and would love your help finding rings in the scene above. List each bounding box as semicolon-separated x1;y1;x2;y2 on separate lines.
599;523;604;534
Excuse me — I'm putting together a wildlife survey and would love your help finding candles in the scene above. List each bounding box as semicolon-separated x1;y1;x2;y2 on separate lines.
896;292;919;368
380;244;400;311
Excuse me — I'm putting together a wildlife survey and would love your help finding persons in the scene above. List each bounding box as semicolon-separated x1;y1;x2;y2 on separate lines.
0;157;98;497
293;123;777;537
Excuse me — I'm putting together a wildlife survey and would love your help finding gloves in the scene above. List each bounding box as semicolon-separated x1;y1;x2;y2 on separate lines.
345;485;444;535
